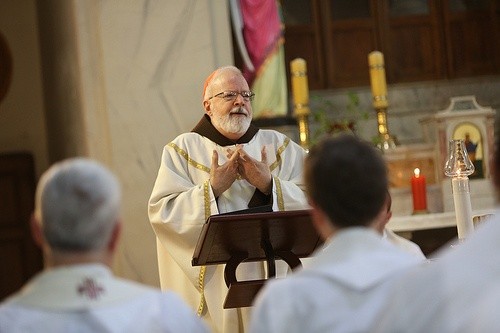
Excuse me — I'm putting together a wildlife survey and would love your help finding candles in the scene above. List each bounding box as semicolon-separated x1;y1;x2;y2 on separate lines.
289;58;309;114
411;168;428;211
368;50;388;108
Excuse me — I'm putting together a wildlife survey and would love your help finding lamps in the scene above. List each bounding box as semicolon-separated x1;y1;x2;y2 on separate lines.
444;139;474;238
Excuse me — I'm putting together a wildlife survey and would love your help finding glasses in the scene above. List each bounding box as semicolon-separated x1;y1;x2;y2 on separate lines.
207;89;255;100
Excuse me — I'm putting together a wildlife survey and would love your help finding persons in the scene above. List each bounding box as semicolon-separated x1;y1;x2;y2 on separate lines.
147;66;329;333
0;134;500;333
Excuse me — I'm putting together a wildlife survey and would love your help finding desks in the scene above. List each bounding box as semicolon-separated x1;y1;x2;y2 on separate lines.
385;210;496;232
